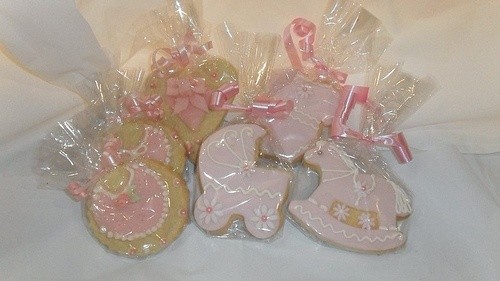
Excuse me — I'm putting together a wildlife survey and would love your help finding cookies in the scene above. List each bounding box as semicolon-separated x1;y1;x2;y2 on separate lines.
79;53;414;259
